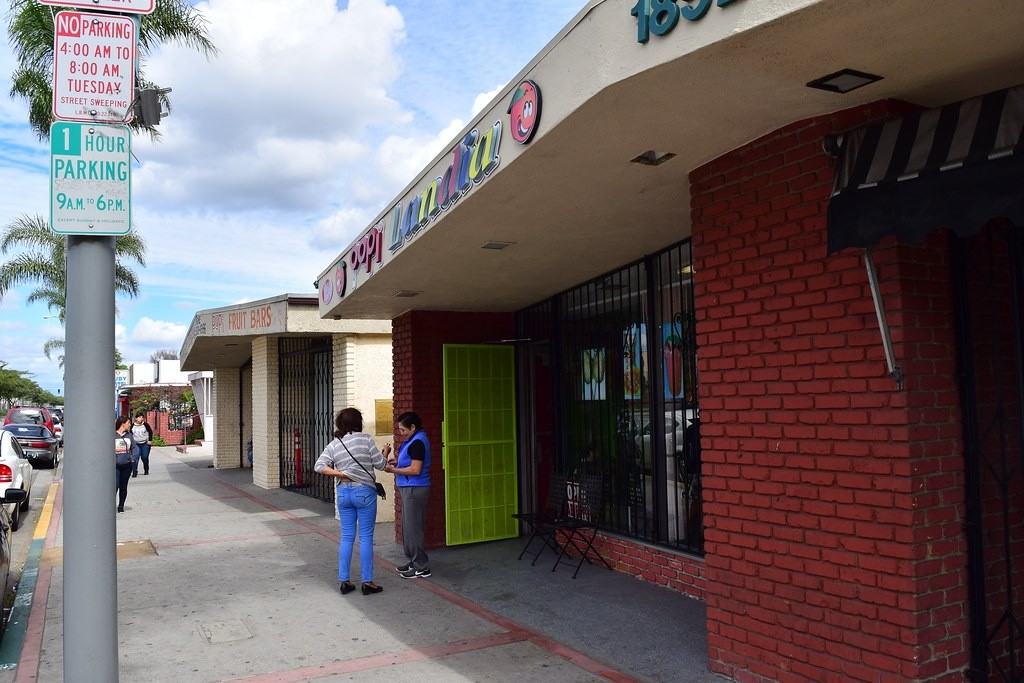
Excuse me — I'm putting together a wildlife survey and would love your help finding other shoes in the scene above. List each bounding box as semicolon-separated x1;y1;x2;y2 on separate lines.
118;506;124;513
143;469;149;475
132;471;138;477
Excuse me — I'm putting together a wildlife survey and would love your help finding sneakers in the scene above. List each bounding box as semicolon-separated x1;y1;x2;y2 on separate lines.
400;567;432;579
396;560;415;572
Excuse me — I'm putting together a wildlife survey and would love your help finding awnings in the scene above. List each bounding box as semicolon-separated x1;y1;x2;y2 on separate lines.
822;86;1024;385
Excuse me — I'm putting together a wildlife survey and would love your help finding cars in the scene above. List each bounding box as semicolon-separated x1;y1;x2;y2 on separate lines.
635;409;699;480
0;430;33;531
52;415;64;446
1;424;60;469
0;488;27;621
56;406;64;413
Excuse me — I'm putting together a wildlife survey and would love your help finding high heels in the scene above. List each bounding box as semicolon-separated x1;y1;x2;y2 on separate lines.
362;583;383;595
340;582;356;595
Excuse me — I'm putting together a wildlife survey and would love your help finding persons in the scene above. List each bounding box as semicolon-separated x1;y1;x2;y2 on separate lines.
386;411;432;578
129;413;153;478
115;415;137;513
313;408;391;596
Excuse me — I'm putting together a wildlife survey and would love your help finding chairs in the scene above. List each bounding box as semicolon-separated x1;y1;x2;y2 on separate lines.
541;472;614;579
512;465;575;566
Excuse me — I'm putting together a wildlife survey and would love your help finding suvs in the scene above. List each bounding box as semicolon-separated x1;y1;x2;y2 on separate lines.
42;408;64;426
3;405;55;435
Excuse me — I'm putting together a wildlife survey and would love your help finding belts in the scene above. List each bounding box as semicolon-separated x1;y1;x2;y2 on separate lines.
339;481;363;487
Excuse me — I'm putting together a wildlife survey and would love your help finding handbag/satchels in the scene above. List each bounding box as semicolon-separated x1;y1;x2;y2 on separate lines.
375;481;388;501
